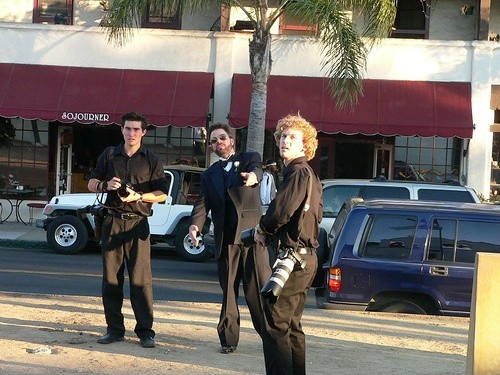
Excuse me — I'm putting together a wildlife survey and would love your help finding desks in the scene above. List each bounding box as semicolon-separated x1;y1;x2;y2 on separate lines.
2;196;27;225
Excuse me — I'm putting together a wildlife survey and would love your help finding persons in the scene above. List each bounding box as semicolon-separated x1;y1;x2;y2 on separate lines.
254;116;323;375
189;122;273;353
88;111;168;347
259;161;279;205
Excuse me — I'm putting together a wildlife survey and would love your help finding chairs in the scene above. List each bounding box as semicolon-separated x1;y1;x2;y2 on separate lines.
27;195;55;226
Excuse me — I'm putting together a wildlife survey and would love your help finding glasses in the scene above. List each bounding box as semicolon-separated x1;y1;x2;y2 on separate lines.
209;134;228;144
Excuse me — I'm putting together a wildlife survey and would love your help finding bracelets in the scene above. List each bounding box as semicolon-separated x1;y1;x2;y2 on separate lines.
96;181;101;190
136;193;142;203
102;181;108;192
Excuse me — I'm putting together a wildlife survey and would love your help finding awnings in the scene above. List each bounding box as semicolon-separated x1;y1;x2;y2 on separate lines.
0;63;213;127
229;74;473;139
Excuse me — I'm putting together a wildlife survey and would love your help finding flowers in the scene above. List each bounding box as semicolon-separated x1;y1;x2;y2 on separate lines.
233;161;241;172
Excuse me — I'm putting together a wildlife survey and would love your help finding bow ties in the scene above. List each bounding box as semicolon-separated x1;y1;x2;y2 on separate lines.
221;154;236;168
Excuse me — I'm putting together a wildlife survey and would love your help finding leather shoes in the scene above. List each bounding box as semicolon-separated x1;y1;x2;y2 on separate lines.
97;333;125;343
140;337;155;347
220;345;236;353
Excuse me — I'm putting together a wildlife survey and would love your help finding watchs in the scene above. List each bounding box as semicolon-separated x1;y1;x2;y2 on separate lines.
255;228;265;235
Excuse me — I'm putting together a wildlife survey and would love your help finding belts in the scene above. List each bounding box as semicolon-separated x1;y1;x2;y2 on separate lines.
108;209;138;220
279;244;316;254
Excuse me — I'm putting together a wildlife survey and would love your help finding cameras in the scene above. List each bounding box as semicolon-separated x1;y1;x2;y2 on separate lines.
240;228;273;248
260;247;306;304
117;177;135;197
